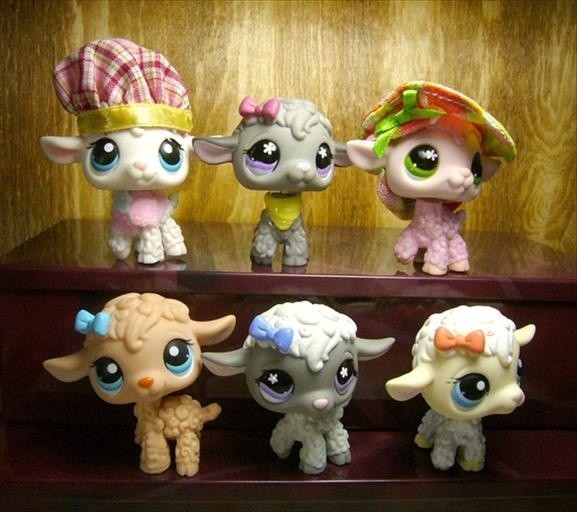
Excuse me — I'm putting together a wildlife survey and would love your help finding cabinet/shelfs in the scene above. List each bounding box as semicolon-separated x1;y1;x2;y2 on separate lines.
1;217;577;506
